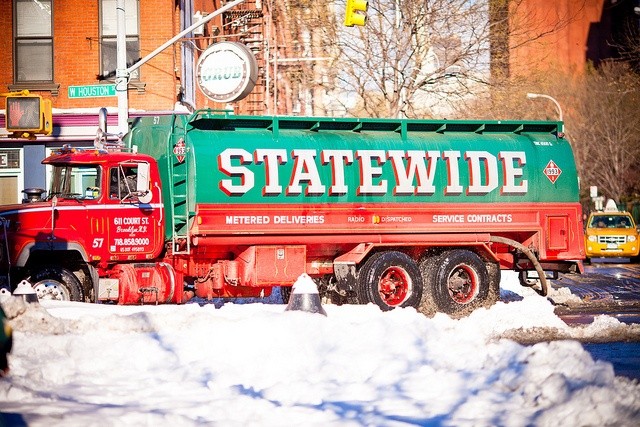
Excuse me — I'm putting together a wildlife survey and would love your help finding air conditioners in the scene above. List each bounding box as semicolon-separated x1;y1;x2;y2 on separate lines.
192;15;204;36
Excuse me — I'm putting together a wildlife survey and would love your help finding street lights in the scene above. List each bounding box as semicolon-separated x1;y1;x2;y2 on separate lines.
526;92;564;136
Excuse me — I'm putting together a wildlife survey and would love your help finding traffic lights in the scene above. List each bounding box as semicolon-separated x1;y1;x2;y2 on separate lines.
346;0;368;28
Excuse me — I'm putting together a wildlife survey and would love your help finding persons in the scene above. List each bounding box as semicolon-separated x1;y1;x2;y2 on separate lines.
616;217;627;228
89;167;137;200
596;219;606;228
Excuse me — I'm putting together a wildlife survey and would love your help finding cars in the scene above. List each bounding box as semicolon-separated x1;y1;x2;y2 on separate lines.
584;210;640;266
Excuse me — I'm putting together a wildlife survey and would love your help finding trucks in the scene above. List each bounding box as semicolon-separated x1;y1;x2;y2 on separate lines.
1;109;586;318
